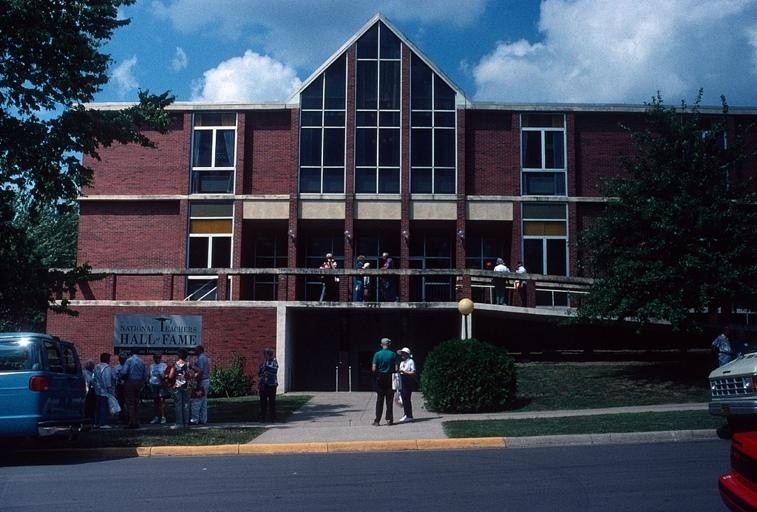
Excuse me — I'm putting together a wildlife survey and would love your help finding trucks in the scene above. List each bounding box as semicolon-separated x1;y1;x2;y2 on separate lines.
708;345;757;416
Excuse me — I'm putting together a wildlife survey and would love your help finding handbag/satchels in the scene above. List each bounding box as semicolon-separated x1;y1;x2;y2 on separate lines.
393;376;404;407
191;386;206;399
84;384;95;412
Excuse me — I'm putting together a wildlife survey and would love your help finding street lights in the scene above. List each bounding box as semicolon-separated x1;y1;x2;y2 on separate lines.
457;298;475;341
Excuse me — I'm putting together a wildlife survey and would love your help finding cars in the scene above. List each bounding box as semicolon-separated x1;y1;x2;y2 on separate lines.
717;429;757;512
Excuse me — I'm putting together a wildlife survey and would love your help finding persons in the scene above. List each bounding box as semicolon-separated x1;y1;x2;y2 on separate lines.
494;258;510;304
320;253;339;301
396;348;415;422
83;345;210;427
372;338;398;424
711;327;732;367
355;255;370;301
258;348;277;421
515;262;527;306
381;252;392;269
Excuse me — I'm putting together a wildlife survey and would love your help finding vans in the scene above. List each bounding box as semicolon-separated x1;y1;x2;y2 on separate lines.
1;331;93;443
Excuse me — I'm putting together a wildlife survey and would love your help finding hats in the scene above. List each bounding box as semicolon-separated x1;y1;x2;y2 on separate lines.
381;338;392;346
396;347;414;359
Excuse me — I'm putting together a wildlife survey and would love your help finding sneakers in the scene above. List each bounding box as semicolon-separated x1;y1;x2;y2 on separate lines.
399;414;413;422
92;422;112;430
169;423;185;430
386;420;393;425
372;422;379;426
188;418;200;425
149;416;168;425
